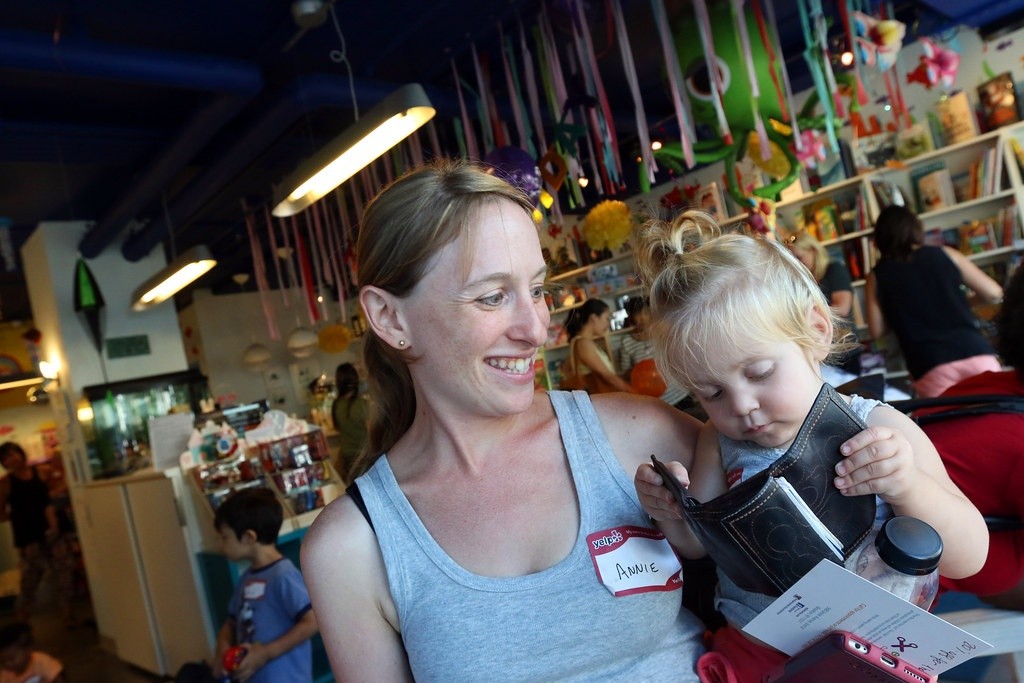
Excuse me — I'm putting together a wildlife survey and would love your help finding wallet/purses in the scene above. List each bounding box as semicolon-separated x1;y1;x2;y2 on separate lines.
651;382;877;599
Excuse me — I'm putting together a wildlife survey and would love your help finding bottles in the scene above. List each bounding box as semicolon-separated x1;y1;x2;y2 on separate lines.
188;420;238;464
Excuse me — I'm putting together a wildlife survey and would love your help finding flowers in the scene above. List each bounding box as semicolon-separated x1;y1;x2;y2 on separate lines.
581;199;636;255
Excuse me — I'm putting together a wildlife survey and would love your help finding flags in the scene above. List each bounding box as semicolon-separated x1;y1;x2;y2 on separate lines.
242;1;914;341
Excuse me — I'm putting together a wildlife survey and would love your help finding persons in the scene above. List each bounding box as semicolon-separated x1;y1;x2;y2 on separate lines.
783;231;861;374
0;442;59;561
331;362;369;469
0;622;65;683
299;158;706;683
906;255;1024;613
633;209;991;683
865;203;1005;399
620;295;696;411
563;298;639;396
211;486;319;683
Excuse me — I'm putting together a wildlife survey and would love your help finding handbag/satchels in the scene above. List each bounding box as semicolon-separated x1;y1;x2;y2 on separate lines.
835;374;1024;533
559;336;617;393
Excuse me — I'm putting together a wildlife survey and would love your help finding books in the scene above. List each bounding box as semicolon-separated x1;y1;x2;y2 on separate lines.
937;90;981;146
971;138;1003;199
911;161;957;212
976;70;1019;130
988;206;1022;248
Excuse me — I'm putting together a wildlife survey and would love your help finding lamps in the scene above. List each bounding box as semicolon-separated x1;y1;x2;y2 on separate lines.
27;361;58;406
288;327;321;358
244;344;271;375
131;243;216;311
269;0;436;218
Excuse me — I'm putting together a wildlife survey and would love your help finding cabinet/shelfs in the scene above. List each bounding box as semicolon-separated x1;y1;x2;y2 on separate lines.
186;422;351;683
539;120;1023;393
84;467;219;678
